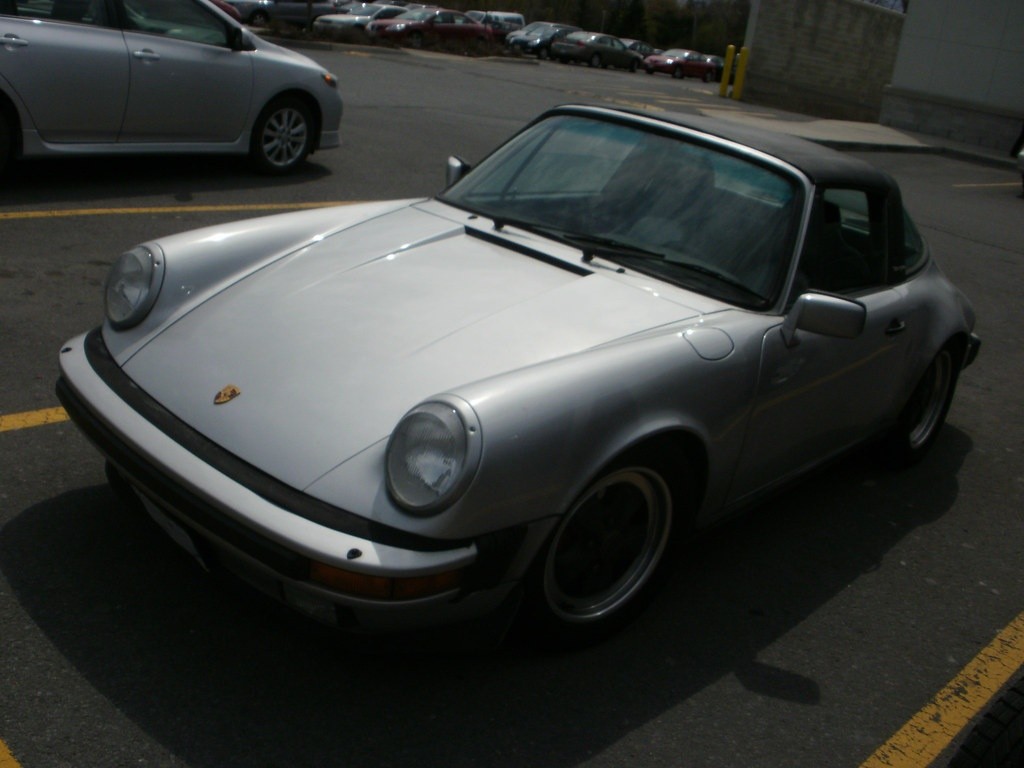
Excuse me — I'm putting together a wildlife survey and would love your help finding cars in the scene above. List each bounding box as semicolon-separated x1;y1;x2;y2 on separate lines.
0;0;343;186
611;38;656;57
705;54;736;85
455;10;526;30
644;48;717;83
54;101;982;651
550;31;643;73
312;4;409;42
144;0;242;26
366;8;494;53
375;0;435;10
226;0;340;28
505;21;583;59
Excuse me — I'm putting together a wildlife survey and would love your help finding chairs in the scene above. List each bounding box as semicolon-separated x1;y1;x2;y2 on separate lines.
50;0;90;22
735;196;848;282
600;153;721;249
